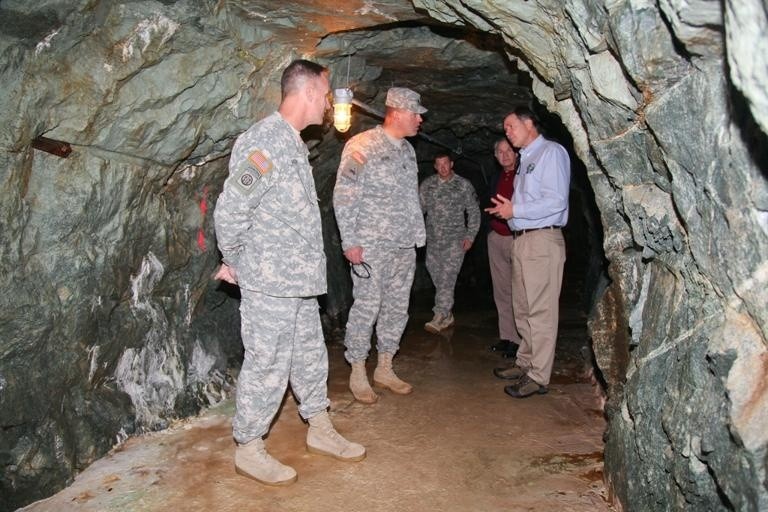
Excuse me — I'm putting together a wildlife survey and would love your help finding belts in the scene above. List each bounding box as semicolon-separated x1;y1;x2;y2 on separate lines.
511;223;563;240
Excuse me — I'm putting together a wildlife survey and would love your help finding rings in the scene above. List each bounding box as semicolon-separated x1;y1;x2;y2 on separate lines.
347;255;352;258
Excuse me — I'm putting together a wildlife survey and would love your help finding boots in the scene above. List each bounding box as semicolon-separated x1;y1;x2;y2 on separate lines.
346;360;379;405
305;409;369;463
372;351;414;395
234;436;298;488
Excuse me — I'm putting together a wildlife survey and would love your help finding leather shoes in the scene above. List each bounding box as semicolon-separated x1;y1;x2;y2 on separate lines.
489;339;519;361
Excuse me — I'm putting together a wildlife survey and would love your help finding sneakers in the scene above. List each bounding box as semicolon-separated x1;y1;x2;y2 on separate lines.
425;313;456;335
504;375;550;402
492;362;525;383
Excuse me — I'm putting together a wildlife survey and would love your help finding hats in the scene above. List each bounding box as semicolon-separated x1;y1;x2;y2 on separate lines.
383;86;428;116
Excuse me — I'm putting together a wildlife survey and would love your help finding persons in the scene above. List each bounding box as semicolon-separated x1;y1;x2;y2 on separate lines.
418;151;482;336
484;106;570;400
206;58;365;485
331;86;428;404
486;137;522;358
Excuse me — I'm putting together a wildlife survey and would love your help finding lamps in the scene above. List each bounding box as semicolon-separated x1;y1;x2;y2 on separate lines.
332;87;354;134
